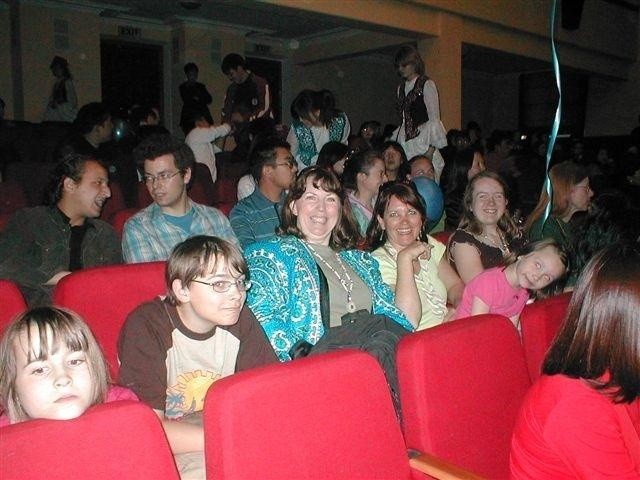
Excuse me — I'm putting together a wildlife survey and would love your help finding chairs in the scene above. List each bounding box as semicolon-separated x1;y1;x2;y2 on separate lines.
54;261;169;383
0;181;28;209
110;208;140;241
430;230;453;243
0;400;181;480
0;208;18;232
100;180;127;224
519;291;574;382
204;349;490;479
215;201;234;214
214;176;242;204
396;314;532;480
0;279;29;345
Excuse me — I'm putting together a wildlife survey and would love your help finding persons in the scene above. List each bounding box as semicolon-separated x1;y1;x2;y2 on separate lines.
451;237;570;331
39;45;640;293
112;234;283;479
240;164;415;365
0;150;123;307
365;178;467;332
509;243;640;480
0;304;141;430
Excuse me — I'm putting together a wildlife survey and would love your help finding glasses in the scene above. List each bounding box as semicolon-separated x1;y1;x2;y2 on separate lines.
140;171;185;185
270;161;299;169
189;277;253;294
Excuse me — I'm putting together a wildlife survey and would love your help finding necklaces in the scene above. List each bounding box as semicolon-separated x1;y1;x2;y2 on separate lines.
303;238;356;315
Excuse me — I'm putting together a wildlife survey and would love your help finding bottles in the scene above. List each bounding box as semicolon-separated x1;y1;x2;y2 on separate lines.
510;208;522;223
